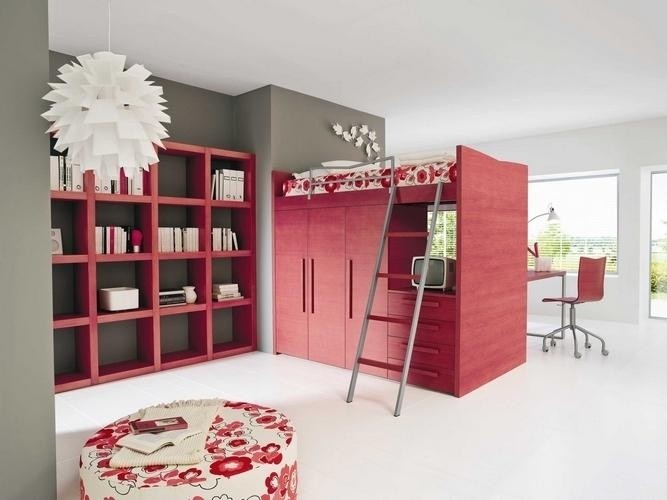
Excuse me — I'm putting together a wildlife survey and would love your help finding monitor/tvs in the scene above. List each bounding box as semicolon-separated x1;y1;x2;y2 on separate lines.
411;256;456;292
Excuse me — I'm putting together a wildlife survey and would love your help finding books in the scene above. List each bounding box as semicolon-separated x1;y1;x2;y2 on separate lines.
50;152;245;308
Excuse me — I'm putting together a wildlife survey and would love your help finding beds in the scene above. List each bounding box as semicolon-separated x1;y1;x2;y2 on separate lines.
273;147;456;196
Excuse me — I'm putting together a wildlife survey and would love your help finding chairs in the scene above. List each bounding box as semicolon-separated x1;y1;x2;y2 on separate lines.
542;257;609;359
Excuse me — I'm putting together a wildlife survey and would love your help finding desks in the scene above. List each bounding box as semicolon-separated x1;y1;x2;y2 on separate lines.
528;270;567;342
78;400;298;500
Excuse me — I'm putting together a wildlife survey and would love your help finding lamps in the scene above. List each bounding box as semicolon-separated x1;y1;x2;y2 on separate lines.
41;0;172;179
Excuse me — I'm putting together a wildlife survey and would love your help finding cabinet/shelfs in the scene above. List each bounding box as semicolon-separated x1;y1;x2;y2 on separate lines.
156;140;206;376
93;142;156;386
272;194;310;360
209;147;260;355
50;124;95;398
389;147;528;397
345;188;388;381
309;192;343;371
